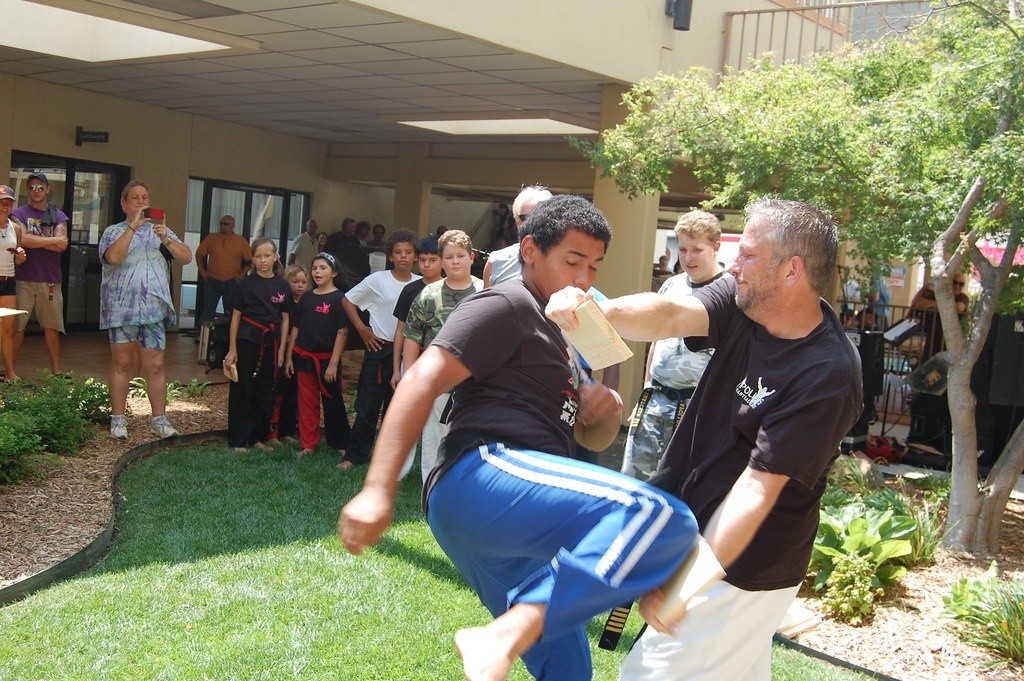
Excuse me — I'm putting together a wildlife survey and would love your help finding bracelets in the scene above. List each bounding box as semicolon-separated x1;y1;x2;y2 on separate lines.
129;225;136;232
165;237;171;246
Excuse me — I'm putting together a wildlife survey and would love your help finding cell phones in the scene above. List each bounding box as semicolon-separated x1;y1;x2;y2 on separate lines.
144;207;165;220
7;247;19;256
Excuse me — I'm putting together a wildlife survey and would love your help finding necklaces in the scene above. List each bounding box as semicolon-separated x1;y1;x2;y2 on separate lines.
0;218;8;237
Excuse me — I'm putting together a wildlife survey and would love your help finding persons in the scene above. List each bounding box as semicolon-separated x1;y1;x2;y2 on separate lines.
335;229;423;467
287;219;386;275
545;198;864;681
437;186;552;278
390;239;443;480
98;180;192;439
0;173;73;384
339;195;699;681
0;184;26;381
224;237;294;452
840;258;969;362
619;212;729;481
653;255;684;278
284;252;350;456
267;265;311;446
195;216;252;345
402;229;486;481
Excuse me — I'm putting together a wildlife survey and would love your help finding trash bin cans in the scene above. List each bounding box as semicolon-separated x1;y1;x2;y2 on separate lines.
838;327;885;456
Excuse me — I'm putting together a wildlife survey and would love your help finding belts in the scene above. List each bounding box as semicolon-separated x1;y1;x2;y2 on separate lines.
0;276;16;280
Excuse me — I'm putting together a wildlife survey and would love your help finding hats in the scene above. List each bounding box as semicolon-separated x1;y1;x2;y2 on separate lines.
0;185;17;202
26;172;47;183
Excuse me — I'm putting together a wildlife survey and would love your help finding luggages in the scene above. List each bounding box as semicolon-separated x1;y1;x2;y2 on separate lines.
197;313;233;366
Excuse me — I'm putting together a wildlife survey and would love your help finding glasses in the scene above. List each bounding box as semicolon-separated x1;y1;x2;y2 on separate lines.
28;184;45;192
516;212;529;222
219;222;231;226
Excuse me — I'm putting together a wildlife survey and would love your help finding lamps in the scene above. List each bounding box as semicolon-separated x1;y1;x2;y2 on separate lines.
665;0;694;30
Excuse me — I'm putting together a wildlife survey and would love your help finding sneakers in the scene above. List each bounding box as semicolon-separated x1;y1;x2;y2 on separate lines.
151;416;179;439
110;414;129;440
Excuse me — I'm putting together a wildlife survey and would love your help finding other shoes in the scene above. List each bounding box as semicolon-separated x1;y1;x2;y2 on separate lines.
0;371;7;381
5;376;33;389
52;374;73;385
